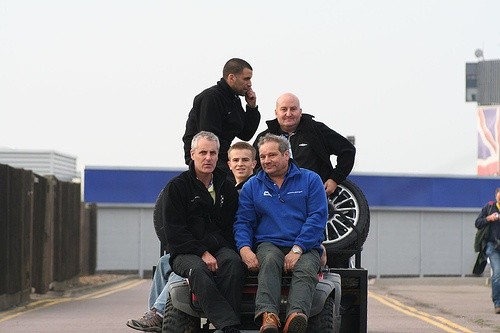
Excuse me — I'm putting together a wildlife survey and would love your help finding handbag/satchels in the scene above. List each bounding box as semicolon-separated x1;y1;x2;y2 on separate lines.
474;225;489;252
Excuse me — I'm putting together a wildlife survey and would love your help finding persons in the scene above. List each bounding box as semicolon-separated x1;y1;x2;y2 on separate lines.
154;131;246;333
472;188;500;314
253;92;356;200
233;133;328;333
125;142;256;331
182;57;261;170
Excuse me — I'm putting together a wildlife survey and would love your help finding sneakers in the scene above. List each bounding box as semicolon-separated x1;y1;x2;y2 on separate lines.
126;308;165;332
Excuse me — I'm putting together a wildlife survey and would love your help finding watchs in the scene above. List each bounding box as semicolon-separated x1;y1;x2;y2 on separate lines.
291;248;302;254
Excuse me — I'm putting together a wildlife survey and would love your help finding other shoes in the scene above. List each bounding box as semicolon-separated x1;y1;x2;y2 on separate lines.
283;312;307;333
495;306;500;314
260;312;280;333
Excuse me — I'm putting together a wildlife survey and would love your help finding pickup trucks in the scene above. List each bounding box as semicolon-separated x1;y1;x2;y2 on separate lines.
153;134;371;333
